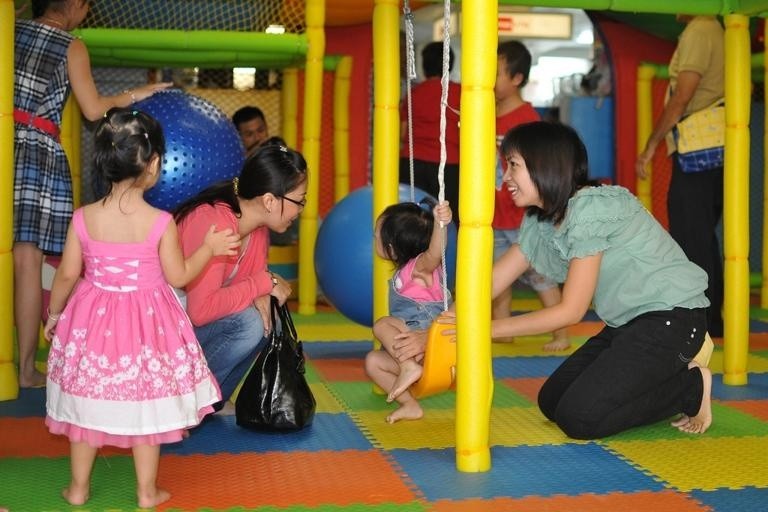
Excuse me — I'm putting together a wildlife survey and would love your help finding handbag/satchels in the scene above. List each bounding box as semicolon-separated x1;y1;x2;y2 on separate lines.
232;291;318;439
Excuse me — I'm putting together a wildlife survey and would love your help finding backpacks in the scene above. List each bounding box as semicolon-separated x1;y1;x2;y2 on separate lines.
665;14;725;171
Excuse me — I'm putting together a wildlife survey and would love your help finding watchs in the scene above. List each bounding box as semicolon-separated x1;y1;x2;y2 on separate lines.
265;271;279;291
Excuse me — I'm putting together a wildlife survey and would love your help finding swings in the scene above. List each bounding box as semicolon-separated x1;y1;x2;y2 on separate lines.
402;0;457;401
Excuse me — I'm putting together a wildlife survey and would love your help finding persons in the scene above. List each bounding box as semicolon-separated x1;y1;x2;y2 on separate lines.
43;107;241;506
11;1;175;388
170;136;309;428
493;39;571;353
400;41;460;222
364;197;455;424
633;13;726;336
391;119;713;439
230;107;268;150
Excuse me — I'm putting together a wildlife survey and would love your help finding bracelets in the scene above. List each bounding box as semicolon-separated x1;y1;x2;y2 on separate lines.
46;309;60;325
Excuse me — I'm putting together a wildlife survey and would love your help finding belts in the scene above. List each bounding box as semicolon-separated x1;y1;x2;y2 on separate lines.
10;106;64;149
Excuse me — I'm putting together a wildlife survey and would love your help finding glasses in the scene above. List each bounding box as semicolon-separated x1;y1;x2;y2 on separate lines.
278;190;308;212
